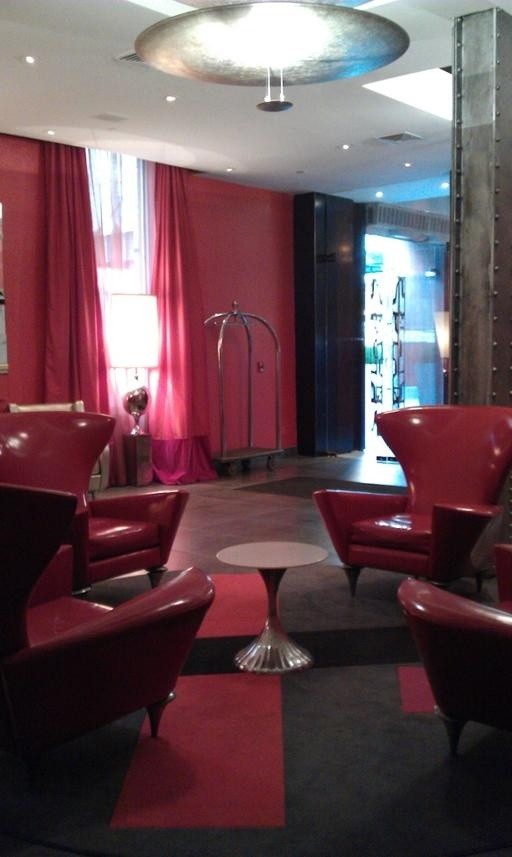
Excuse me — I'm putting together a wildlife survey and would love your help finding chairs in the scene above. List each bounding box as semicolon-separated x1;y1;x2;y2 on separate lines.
9;400;110;501
314;404;512;597
0;409;189;593
394;543;512;767
0;528;216;789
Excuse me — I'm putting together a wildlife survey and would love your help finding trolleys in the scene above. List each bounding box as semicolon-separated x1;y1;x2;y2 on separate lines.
204;298;287;476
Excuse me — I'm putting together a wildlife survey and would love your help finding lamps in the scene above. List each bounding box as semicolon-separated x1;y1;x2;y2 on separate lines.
134;0;409;111
433;311;451;372
